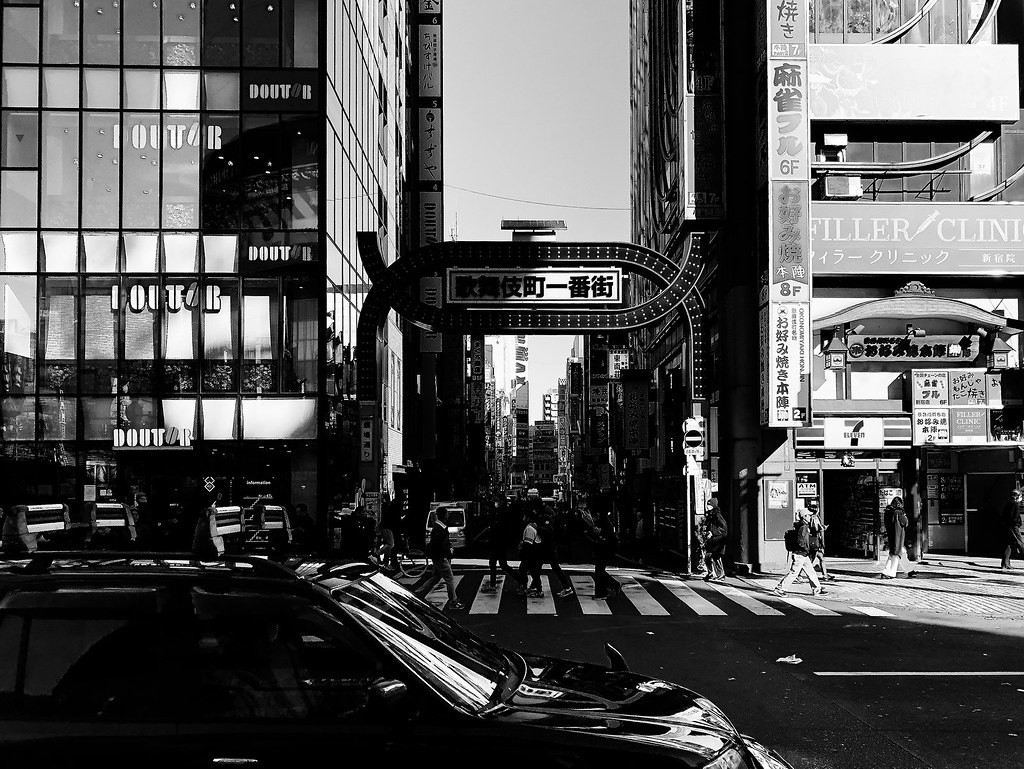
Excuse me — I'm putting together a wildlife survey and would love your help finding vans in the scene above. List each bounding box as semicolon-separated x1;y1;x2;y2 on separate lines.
425;506;472;549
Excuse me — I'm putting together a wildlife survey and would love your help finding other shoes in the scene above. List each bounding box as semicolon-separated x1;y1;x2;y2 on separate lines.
713;575;725;581
823;574;835;581
449;600;465;610
813;589;829;596
882;574;891;580
558;587;574;597
908;570;918;579
481;586;496;593
517;585;526;594
593;595;607;600
524;588;543;598
1002;564;1014;570
614;583;623;596
774;587;788;597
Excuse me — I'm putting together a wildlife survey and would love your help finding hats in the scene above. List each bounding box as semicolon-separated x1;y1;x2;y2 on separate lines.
707;498;718;508
799;509;814;517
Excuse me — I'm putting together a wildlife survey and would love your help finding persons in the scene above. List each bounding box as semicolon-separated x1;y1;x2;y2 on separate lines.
773;504;835;598
191;508;223;560
251;500;266;529
293;503;316;556
881;497;918;579
341;494;405;565
481;497;574;597
589;513;621;600
414;507;464;609
704;498;728;581
211;617;325;719
1000;490;1024;569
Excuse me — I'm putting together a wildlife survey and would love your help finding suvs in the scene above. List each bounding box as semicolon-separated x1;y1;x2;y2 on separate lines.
0;550;801;769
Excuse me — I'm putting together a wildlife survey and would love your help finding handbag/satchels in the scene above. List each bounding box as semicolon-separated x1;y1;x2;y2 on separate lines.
784;529;800;551
809;536;819;550
